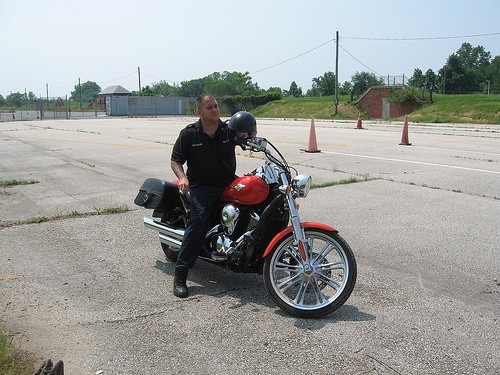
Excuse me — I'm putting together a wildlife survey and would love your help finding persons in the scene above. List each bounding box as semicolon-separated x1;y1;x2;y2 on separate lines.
170;94;248;297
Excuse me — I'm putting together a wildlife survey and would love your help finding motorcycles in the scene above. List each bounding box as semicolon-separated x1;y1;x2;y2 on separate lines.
134;137;357;318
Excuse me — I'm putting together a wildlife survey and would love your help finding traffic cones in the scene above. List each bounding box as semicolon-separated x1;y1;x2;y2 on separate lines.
305;118;321;153
398;115;412;146
354;112;364;129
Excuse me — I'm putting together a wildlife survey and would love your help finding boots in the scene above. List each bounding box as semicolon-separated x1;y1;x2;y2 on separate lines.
174;265;190;297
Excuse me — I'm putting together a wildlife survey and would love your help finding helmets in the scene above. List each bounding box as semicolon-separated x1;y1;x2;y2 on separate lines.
228;111;257;132
